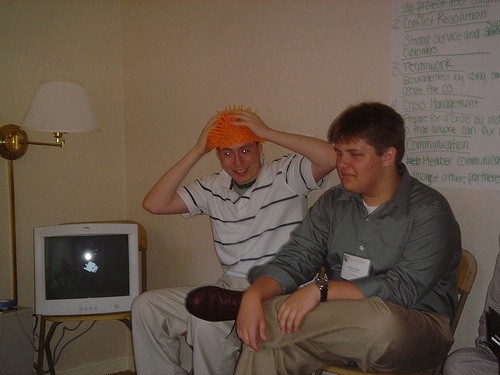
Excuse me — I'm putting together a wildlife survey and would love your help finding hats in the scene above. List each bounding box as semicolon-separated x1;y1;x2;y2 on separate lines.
205;104;266;149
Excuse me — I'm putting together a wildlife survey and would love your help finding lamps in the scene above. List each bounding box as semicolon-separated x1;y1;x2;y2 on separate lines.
0;81;103;306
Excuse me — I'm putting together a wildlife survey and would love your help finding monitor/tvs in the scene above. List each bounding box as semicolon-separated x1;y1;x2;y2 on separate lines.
33;221;143;316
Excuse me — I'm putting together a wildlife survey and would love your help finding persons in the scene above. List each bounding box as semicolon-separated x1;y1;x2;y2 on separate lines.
185;98;462;375
129;106;338;375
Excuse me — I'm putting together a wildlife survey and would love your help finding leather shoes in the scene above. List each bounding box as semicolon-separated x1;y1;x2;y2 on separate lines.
185;286;245;322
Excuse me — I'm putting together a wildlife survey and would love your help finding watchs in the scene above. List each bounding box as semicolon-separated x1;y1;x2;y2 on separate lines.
313;275;329;303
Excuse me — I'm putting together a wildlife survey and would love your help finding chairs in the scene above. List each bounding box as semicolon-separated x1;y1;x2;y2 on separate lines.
314;249;477;375
38;221;146;375
